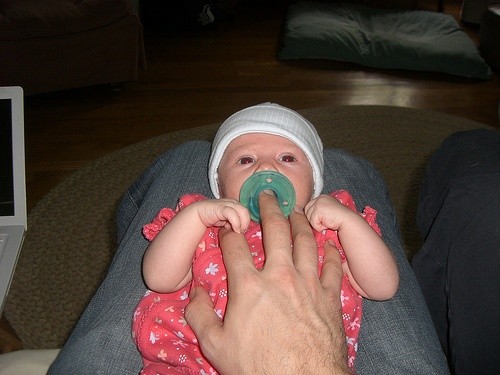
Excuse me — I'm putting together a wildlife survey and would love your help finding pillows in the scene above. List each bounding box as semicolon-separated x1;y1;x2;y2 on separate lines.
274;3;494;81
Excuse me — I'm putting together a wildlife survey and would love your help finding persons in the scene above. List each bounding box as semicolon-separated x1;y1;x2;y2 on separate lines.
46;138;453;375
411;127;500;374
130;101;401;375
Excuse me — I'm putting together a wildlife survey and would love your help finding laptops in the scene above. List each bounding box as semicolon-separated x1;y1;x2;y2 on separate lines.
0;86;28;316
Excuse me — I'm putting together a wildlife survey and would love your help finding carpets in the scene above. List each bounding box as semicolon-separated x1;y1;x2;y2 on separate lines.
5;104;494;350
